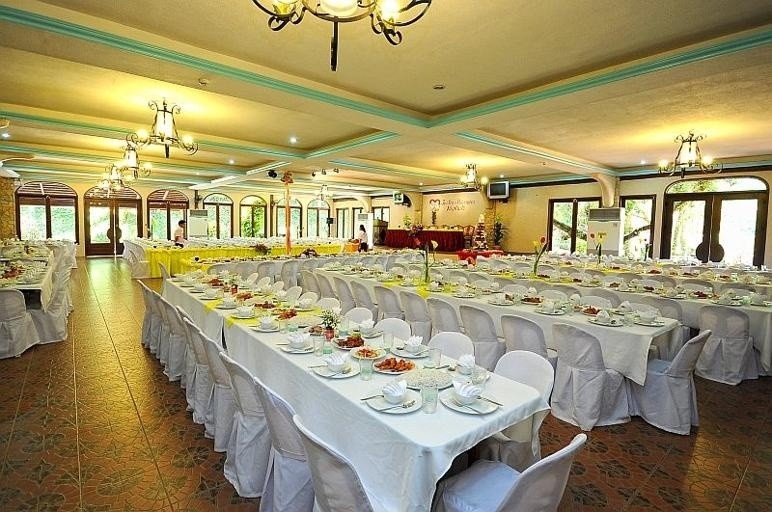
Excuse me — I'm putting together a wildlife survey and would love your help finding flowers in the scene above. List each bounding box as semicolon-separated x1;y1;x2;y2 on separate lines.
533;236;548;276
590;234;602;263
414;237;438;282
317;310;341;329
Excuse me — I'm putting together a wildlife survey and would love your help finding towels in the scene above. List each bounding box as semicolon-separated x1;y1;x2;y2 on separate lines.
492;265;499;275
528;286;537;295
515;268;527;277
333;306;342;317
226;297;235;306
457;275;469;284
597;310;611;323
583;273;600;285
429;281;437;290
542;300;556;312
298;298;312;309
359;319;376;334
492;280;501;291
371;262;383;271
750;294;769;308
444;259;459;266
324;352;348;370
260;287;272;293
431;270;443;282
667;288;676;297
499;294;510;306
403;335;423;352
452;382;480;404
382;381;407;404
641;309;658;323
220;270;229;279
186;278;194;285
460;288;471;298
619;282;631;290
570;292;581;304
287;335;310;347
409;267;422;280
195;284;207;291
362;270;372;278
323;260;336;271
379;273;395;283
459;354;477;376
400;277;414;285
276;290;288;299
389;265;404;275
344;267;355;273
468;265;478;273
619;299;633;313
205;291;216;297
550;274;563;283
242;281;251;287
355;258;364;266
718;296;730;305
175;272;184;279
237;306;254;316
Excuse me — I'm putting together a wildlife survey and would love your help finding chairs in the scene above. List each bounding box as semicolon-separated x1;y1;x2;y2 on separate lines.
253;377;315;512
292;414;386;512
297;259;319;283
425;297;464;339
298;291;317;301
682;278;714;293
468;272;488;284
459;304;506;373
183;318;213;424
478;350;555;472
374;317;412;339
325;254;422;273
584;269;606;278
399;290;432;345
579;296;612;309
630;303;661;317
317;298;340;310
345;307;373;324
430;268;443;279
428;332;474;360
526;281;551;293
513;263;530;268
235;262;256;275
550;323;631;432
501;314;557;372
275;260;297;290
391;262;406;273
552;285;582;297
160;296;186;382
286;286;302;299
647;276;676;287
218;353;272;497
0;289;40;360
720;283;756;293
299;270;321;299
630;329;712;435
259;276;270;286
137;279;153;349
662;264;680;269
175;305;198;412
689;267;713;274
640;296;690;344
587;288;622;308
476;280;489;287
198;332;237;453
273;281;284;291
632;262;649;266
560;267;580;276
476;259;486;263
450;271;466;282
127;248;149;280
257;261;275;284
538;264;555;271
432;433;587;512
374;286;405;321
695;306;767;386
617;273;643;283
409;265;421;276
493;259;507;266
540;290;568;301
333;277;356;315
314;272;339;300
158;262;171;295
207;263;230;275
122;240;144;261
27;243;78;344
350;280;377;321
494;277;517;287
502;284;527;293
153;290;170;366
247;272;259;285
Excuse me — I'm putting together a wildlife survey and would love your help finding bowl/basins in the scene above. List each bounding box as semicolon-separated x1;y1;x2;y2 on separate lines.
403;343;421;353
456;364;475;374
327;362;351;372
382;393;407;403
452;392;477;404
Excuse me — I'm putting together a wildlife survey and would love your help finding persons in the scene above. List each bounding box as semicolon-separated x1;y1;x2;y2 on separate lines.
358;225;368;252
174;220;187;248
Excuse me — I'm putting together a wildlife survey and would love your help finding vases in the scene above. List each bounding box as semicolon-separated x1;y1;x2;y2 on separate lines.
326;329;334;340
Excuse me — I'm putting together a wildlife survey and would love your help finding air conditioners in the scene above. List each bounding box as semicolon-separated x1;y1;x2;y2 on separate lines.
187;209;208;240
358;213;373;249
587;208;625;255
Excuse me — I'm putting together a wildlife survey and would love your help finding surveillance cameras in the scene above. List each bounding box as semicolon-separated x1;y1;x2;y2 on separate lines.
268;170;278;179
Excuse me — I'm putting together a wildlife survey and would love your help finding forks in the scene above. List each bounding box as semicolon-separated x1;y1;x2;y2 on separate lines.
448;394;482;418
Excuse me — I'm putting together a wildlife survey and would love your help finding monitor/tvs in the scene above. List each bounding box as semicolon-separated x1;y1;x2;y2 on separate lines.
487;180;509;199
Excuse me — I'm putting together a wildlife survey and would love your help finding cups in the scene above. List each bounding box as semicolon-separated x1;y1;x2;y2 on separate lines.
443;282;452;293
184;277;192;285
429;348;441;366
195;284;203;290
239;306;251;317
471;368;488;392
490;282;499;291
358;323;373;334
640;312;657;323
421;384;438;413
718;294;732;305
752;293;767;304
618;301;633;312
622;312;634;327
460;289;469;296
279;319;288;334
358;357;374;382
511;291;523;306
341;316;349;329
565;303;574;316
223;298;234;307
475;288;483;299
299;299;312;308
383;332;394;347
666;287;678;297
527;287;538;296
430;281;439;290
206;289;216;298
313;337;325;356
569;293;581;306
596;309;610;324
494;293;506;304
541;301;554;313
289;334;308;349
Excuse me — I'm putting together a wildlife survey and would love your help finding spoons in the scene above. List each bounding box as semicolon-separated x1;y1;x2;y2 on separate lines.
281;346;319;355
330;367;353;380
377;400;416;413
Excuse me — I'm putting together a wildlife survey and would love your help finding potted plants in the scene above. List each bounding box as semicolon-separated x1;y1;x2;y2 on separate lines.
491;214;504;250
402;216;411;228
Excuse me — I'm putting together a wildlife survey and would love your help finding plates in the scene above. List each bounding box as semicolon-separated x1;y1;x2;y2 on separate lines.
390;343;429;359
288;306;313;312
214;304;238;309
231;314;254;319
312;357;361;378
199;295;218;300
252;326;280;333
588;316;623;326
189;289;205;293
534;307;565;315
397;368;455;389
487;298;513;306
366;388;423;413
424;288;443;292
448;364;490;382
660;294;687;299
180;283;195;287
454;293;474;298
347;328;382;338
373;358;416;374
710;299;742;306
439;386;499;414
351;346;386;360
635;318;664;327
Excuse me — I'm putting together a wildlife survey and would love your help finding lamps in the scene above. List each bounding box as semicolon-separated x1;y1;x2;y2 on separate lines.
98;144;152;198
460;163;481;189
253;0;431;72
126;98;198;158
658;129;723;178
